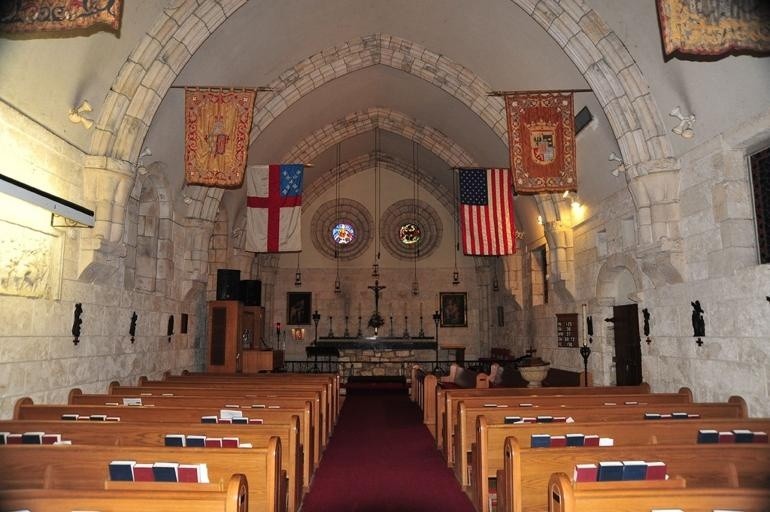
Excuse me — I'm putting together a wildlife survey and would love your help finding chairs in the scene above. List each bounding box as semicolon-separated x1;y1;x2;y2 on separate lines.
476;348;511;389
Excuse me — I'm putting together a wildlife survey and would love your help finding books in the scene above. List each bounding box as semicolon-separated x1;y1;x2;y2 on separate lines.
61;414;120;422
225;404;281;408
645;413;700;419
201;416;264;424
574;460;667;481
504;416;567;423
531;434;613;447
0;431;72;445
165;434;254;449
484;404;533;407
105;402;155;406
698;429;770;444
109;460;208;483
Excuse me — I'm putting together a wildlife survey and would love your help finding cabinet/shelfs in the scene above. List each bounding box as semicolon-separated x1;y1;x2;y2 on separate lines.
208;300;266;374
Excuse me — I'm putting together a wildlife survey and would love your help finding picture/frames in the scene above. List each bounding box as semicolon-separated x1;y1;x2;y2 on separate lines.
439;292;468;328
286;291;312;326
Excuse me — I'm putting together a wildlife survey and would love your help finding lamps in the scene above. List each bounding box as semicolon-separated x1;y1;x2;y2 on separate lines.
70;100;95;129
138;147;153;174
608;152;626;176
669;106;696;140
294;129;502;295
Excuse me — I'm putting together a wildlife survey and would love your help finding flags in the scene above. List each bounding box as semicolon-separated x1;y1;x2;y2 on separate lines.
458;168;517;257
505;90;578;194
244;162;304;253
654;1;770;64
0;0;124;41
184;84;257;189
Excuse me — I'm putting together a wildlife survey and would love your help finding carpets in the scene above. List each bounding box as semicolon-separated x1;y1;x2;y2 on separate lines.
302;382;476;512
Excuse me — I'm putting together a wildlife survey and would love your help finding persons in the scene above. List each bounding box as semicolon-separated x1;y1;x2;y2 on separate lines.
445;296;463;325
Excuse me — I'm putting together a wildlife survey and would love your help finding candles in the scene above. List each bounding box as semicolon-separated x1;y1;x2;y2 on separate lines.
314;293;440;318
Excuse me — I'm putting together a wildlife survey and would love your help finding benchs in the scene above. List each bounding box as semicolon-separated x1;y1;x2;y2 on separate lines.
434;381;770;512
0;370;339;512
